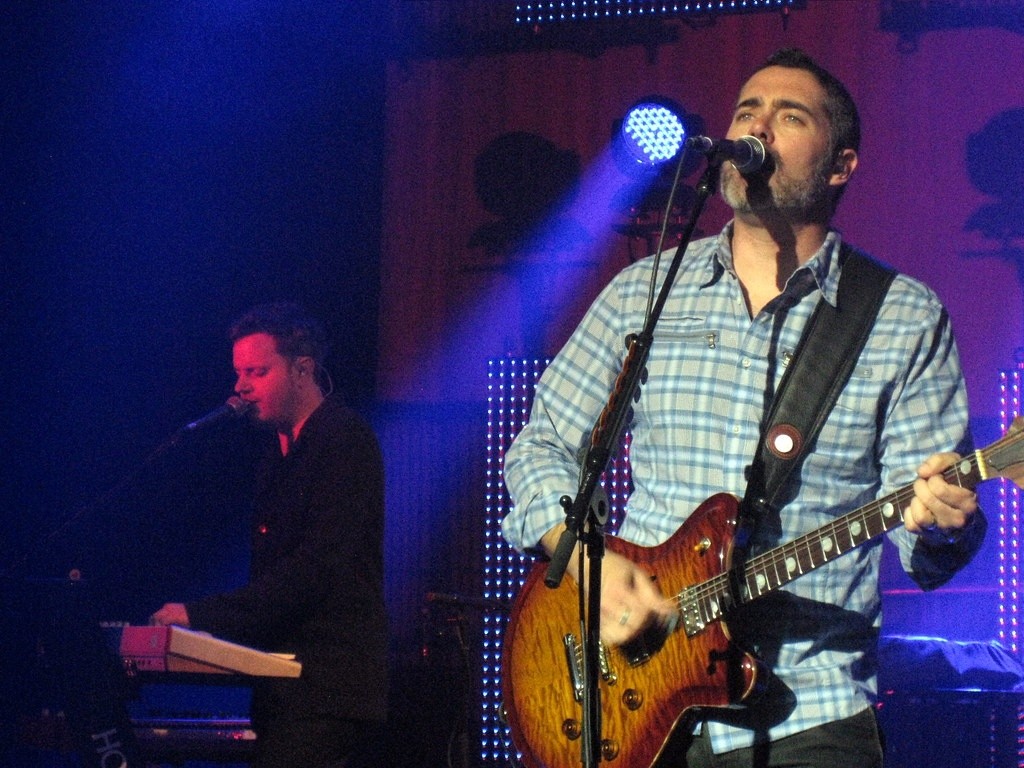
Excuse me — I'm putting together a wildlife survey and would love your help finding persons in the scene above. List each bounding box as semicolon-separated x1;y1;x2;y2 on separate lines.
148;312;389;768
501;47;987;768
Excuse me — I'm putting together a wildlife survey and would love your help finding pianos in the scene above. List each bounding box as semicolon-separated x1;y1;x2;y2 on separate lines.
96;621;303;680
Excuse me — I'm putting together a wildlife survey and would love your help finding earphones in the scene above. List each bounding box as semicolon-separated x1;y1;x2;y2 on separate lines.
298;367;308;375
833;156;851;172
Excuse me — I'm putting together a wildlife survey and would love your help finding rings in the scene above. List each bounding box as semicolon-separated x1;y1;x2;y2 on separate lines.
619;609;630;626
927;515;936;531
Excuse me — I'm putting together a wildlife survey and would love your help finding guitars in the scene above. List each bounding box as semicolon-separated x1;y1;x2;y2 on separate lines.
500;414;1024;768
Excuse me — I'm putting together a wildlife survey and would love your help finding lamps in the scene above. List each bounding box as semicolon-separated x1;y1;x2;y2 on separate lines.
607;95;706;185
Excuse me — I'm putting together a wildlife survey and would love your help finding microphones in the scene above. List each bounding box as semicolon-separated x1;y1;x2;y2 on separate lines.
176;396;249;436
686;135;768;174
